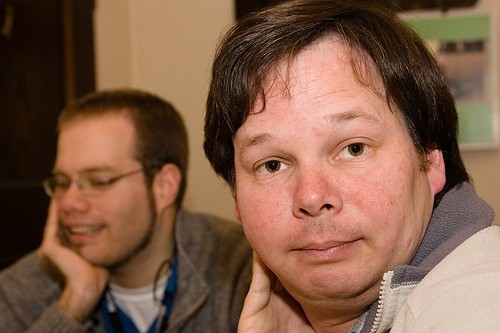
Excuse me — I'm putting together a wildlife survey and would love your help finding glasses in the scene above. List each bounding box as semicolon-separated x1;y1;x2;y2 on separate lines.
42;167;150;196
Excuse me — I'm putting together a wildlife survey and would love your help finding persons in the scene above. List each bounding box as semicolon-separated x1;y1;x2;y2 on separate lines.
203;0;500;333
0;89;253;333
